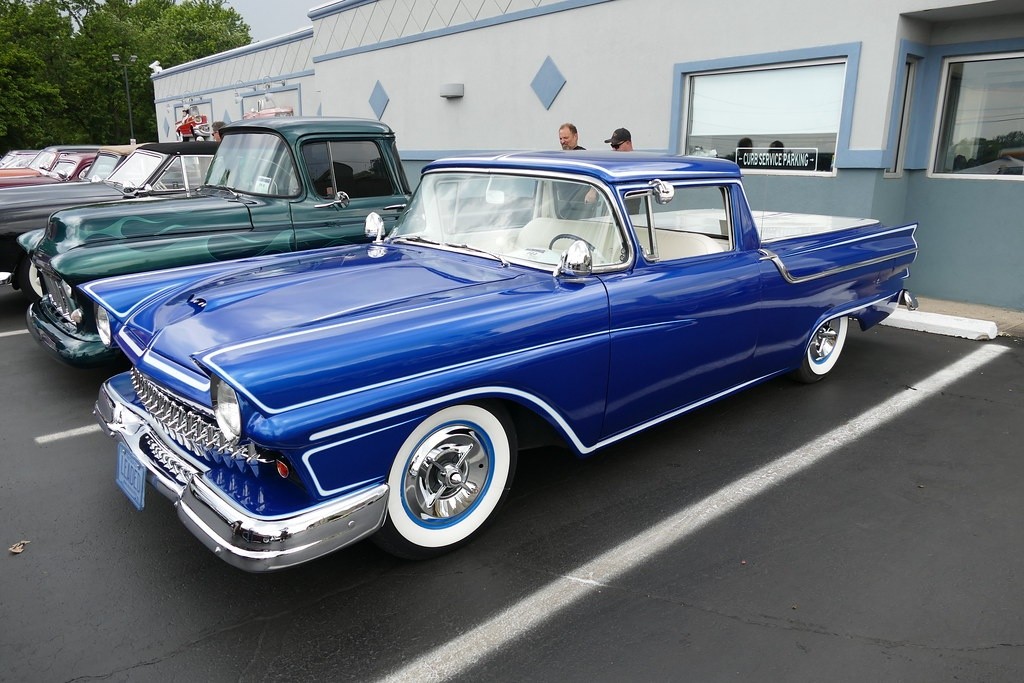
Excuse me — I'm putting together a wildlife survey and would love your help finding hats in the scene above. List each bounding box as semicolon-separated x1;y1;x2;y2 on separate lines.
604;128;631;143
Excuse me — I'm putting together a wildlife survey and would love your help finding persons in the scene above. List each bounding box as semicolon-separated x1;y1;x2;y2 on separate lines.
173;109;192;142
210;120;227;141
726;137;790;165
552;121;600;224
602;127;643;216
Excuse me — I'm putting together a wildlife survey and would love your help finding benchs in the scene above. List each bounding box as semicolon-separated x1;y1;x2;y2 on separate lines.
516;218;724;263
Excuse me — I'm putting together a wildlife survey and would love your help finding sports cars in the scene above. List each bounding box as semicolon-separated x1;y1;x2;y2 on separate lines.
92;149;919;575
0;116;593;370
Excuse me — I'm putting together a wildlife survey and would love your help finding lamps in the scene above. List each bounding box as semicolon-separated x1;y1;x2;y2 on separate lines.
165;93;185;113
262;75;286;102
182;90;202;110
234;79;257;104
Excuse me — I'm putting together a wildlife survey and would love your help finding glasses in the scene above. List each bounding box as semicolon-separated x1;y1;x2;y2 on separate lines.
611;140;626;149
212;132;215;136
